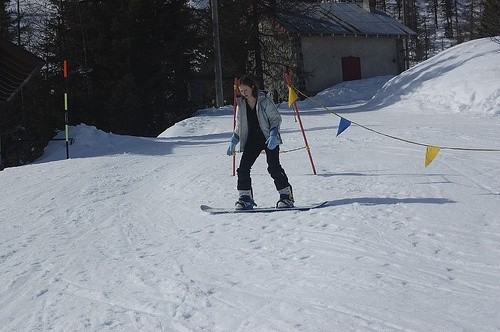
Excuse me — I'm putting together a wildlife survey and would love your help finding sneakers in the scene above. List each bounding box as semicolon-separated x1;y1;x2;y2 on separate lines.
235;188;255;210
275;185;294;209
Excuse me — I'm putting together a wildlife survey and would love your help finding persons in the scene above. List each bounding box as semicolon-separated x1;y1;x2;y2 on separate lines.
226;77;297;209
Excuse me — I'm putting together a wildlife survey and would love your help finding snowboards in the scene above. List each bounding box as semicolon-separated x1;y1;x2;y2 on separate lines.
200;201;327;213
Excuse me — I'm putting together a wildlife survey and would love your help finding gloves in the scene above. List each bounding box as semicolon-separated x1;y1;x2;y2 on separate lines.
226;133;240;156
265;128;279;151
